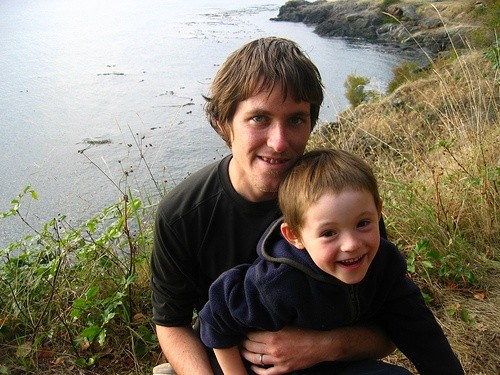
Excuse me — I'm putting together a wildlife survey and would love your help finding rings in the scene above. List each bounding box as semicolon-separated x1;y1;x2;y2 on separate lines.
259;354;265;366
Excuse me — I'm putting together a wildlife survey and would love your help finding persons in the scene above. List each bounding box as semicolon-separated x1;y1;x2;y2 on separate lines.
150;37;388;375
197;151;463;375
